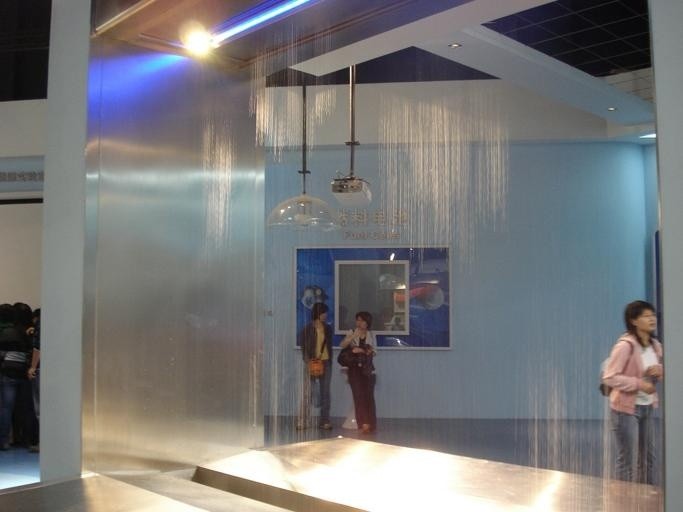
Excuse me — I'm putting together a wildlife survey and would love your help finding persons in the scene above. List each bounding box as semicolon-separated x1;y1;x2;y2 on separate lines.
599;297;666;486
337;310;379;435
294;302;335;431
0;301;40;452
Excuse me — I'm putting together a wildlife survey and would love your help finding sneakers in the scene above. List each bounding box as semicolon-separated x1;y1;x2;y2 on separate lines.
352;426;376;435
319;422;332;430
295;422;312;431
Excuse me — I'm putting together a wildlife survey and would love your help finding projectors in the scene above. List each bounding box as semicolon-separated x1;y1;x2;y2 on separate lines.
331;178;372;207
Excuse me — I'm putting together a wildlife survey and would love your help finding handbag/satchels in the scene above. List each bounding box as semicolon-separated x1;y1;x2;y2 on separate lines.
337;343;373;377
599;382;613;396
307;356;325;376
